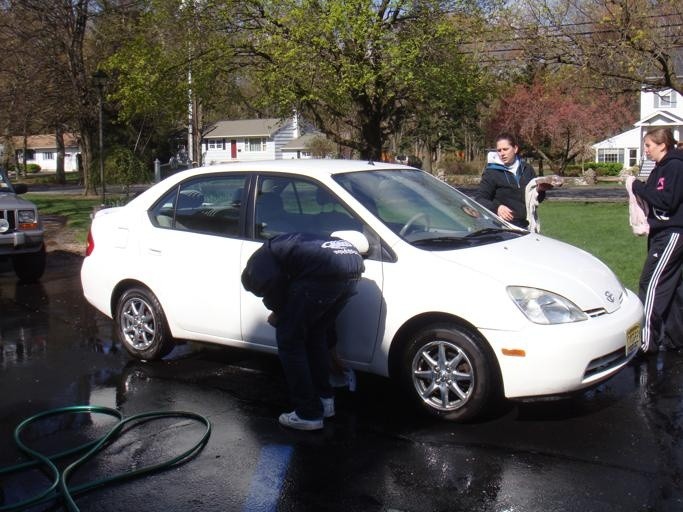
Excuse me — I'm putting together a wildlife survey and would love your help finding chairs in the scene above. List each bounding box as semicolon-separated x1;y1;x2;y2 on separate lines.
162;185;354;239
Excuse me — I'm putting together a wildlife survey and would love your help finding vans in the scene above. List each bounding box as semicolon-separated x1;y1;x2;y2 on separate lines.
394;155;423;170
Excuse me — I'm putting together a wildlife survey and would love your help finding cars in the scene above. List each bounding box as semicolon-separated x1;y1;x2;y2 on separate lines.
81;158;646;424
0;168;45;281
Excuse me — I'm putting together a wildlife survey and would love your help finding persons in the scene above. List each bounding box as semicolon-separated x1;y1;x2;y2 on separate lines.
628;128;682;356
240;233;365;431
378;155;425;236
474;132;554;235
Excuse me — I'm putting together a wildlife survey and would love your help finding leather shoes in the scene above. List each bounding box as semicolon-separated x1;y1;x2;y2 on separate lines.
656;342;681;353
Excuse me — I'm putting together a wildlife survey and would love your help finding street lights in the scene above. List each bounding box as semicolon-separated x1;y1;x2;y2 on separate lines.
93;69;107;204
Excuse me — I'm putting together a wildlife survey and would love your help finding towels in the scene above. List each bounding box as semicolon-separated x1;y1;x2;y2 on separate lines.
626;177;650;236
329;362;356;393
525;170;563;233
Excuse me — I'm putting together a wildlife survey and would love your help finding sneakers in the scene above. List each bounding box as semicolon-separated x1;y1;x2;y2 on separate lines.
278;410;324;431
318;395;336;419
